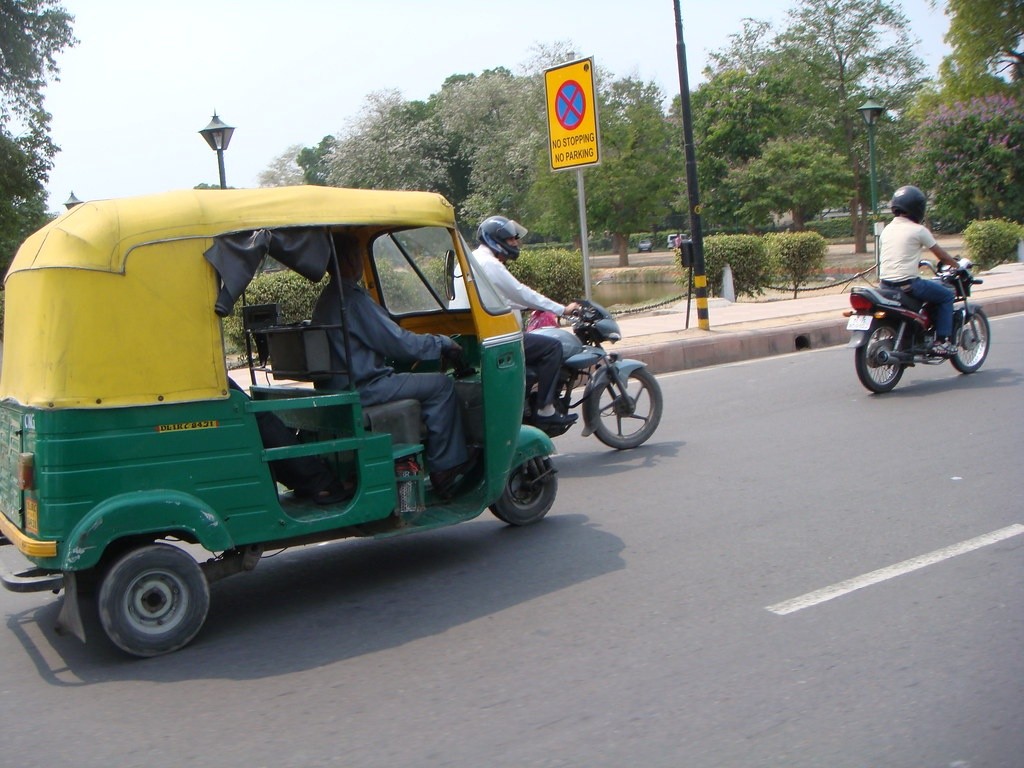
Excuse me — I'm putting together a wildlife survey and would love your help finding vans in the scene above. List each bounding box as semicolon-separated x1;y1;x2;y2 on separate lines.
667;234;689;250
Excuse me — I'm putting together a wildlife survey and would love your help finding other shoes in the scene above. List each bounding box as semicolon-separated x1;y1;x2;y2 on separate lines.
430;449;484;499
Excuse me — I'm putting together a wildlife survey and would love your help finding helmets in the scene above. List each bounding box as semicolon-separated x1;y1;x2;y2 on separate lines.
890;185;927;224
477;215;529;261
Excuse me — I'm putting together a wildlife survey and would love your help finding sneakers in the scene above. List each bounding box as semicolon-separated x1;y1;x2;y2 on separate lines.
930;337;958;358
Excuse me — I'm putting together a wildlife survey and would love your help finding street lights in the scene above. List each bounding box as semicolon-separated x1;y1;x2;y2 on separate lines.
197;106;257;385
61;190;84;211
857;96;885;280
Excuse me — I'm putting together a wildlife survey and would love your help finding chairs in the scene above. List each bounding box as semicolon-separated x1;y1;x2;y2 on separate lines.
266;325;421;445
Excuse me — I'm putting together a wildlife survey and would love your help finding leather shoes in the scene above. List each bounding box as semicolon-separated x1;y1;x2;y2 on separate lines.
535;410;579;429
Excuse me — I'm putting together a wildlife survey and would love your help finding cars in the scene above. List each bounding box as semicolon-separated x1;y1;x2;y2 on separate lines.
638;240;652;253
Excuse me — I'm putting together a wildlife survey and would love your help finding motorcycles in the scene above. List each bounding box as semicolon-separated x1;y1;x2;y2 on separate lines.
521;299;663;450
843;258;991;394
1;187;556;656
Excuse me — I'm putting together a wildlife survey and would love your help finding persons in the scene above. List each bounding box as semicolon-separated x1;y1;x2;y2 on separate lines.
309;233;478;492
450;216;580;428
227;376;341;504
687;234;691;240
879;186;960;356
675;234;681;247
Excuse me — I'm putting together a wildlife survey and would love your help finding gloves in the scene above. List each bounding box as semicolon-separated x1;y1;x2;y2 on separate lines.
957;258;973;271
